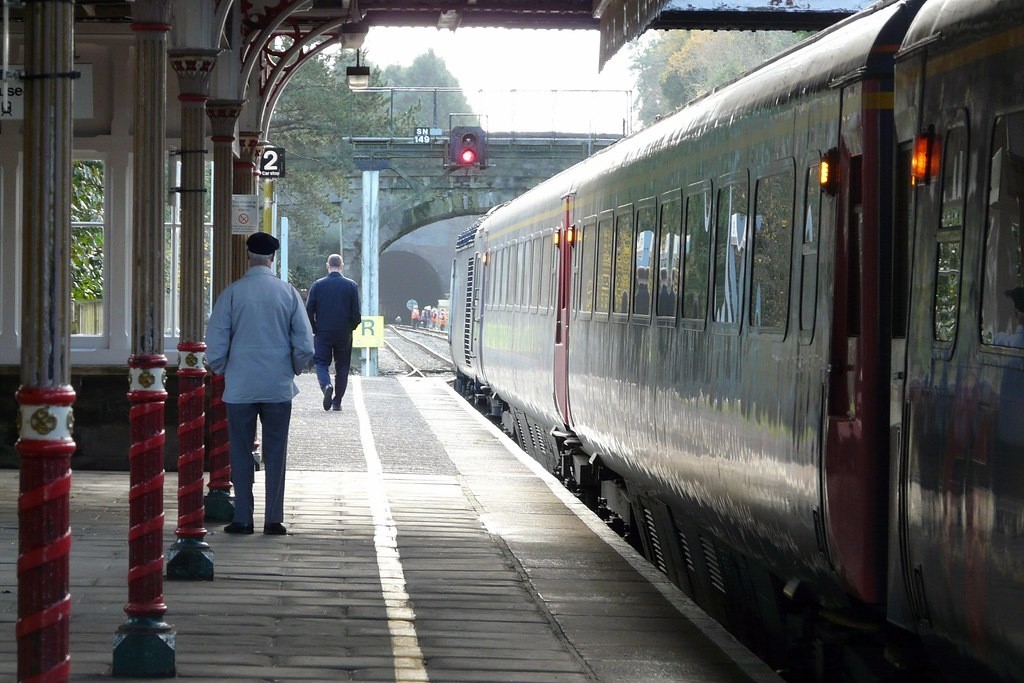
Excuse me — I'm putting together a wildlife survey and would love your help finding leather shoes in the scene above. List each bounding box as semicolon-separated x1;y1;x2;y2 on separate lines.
333;405;342;411
265;522;286;535
224;521;254;534
323;383;333;412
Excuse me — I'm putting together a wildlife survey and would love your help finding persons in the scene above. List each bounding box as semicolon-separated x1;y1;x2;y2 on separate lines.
421;307;446;331
204;232;314;535
412;305;420;330
306;254;361;410
620;263;676;316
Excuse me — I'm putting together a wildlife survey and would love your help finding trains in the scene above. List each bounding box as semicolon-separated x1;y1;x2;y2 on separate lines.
448;0;1024;683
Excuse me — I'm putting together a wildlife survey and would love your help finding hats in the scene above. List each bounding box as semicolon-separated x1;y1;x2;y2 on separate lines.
246;232;280;256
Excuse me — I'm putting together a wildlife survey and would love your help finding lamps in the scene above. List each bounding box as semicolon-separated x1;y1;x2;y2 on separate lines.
346;49;370;90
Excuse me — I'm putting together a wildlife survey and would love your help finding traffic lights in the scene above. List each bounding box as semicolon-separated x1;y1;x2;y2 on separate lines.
451;126;486;165
